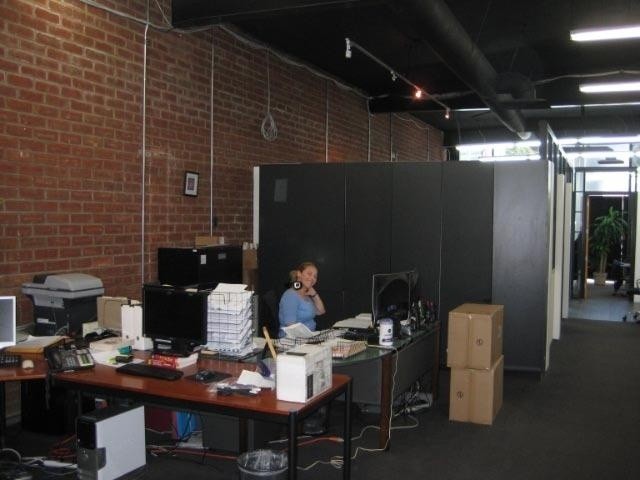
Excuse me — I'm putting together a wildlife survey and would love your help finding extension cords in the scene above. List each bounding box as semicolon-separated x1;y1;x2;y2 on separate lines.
401;400;431;412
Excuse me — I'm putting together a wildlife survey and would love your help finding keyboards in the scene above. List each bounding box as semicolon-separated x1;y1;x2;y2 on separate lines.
118;363;183;382
1;354;21;368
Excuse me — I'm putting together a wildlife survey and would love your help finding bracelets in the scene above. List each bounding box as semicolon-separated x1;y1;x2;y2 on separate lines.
311;292;317;297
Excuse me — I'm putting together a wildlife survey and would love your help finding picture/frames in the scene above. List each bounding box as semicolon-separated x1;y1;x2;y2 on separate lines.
183;170;200;196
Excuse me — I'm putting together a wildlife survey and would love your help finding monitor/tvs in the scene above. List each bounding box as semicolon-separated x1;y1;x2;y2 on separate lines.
372;268;420;329
0;296;17;350
140;283;212;355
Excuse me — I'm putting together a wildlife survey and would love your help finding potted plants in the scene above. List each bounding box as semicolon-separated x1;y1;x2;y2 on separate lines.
588;205;630;290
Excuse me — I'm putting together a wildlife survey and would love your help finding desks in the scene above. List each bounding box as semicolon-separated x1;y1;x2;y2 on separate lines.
241;320;441;451
0;329;354;480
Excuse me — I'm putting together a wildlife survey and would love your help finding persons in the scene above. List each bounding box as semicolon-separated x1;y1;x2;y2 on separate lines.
277;261;326;341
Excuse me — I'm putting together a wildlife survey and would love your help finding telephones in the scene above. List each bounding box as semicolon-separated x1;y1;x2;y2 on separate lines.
46;347;95;371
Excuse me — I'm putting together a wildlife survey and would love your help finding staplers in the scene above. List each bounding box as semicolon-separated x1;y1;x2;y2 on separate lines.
256;360;270;377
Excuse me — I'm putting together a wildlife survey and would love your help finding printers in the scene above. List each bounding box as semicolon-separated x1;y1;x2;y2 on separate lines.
23;272;106;333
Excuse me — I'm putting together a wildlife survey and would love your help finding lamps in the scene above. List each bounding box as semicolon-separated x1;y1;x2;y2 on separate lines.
516;127;534;141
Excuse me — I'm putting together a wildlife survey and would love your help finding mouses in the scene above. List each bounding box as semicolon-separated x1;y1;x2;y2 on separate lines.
194;369;212;381
23;360;35;370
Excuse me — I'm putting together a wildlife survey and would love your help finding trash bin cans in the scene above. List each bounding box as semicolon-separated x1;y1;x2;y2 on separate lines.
235;449;289;480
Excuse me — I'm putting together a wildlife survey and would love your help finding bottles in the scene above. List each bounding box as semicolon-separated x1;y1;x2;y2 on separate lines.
410;297;439;331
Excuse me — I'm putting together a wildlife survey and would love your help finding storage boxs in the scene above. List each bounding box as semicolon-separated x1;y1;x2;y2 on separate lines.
449;355;505;425
447;303;504;370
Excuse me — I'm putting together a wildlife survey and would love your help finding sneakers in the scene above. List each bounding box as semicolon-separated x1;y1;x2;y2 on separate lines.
303;423;324;435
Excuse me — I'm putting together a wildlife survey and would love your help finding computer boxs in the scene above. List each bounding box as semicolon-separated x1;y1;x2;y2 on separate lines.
76;399;149;479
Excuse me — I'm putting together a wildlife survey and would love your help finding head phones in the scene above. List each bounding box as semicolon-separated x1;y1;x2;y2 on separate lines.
292;280;302;291
